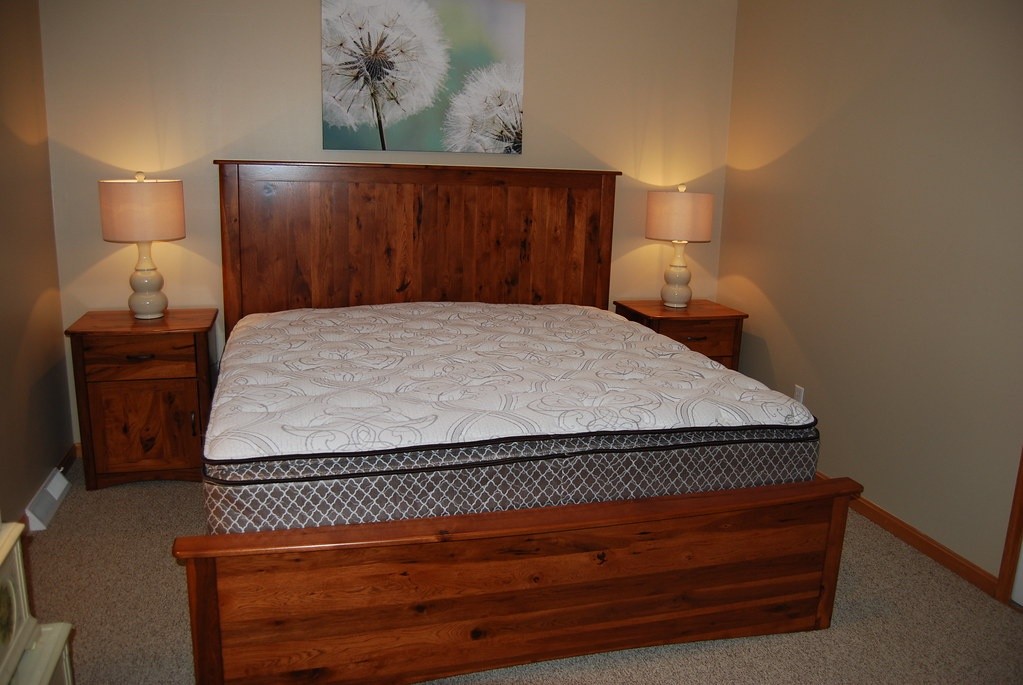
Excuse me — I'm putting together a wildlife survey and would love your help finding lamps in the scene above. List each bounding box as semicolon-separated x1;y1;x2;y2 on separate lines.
98;171;186;320
644;183;715;309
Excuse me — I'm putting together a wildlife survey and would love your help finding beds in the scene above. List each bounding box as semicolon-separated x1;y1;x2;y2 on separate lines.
172;157;865;685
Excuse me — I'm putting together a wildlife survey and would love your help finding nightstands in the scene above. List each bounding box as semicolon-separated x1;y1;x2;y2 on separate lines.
64;310;219;490
613;299;750;373
